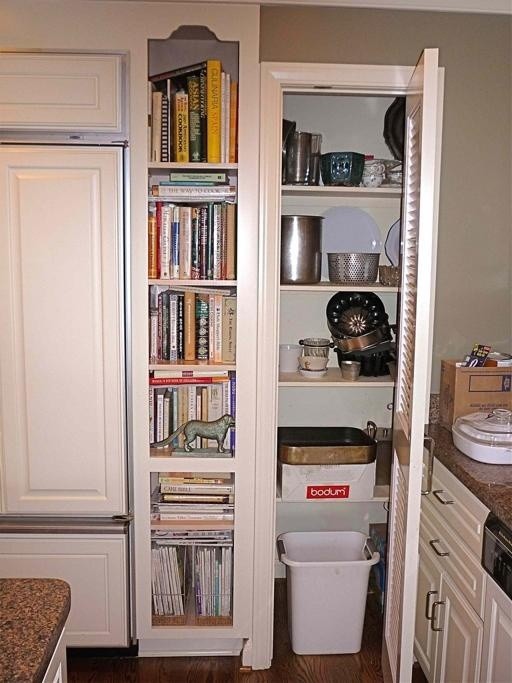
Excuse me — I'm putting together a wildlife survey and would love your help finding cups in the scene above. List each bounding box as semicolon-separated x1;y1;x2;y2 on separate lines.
362;160;385;189
341;361;361;380
285;131;313;185
279;344;304;373
309;134;322;186
281;214;326;284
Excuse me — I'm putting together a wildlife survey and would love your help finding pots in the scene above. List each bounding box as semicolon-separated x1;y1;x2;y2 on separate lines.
329;319;397;353
331;347;392;377
325;292;385;339
450;409;512;464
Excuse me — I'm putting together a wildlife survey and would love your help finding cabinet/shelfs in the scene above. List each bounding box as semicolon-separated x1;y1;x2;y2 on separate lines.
132;0;264;673
478;573;512;683
266;48;447;683
414;446;484;683
2;50;129;648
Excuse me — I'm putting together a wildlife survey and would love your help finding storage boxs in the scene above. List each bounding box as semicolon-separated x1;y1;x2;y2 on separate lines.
278;461;376;500
439;359;512;432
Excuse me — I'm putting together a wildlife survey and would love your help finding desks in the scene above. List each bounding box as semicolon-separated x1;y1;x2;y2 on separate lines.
2;579;75;682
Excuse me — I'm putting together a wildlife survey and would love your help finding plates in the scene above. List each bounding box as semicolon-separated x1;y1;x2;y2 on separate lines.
384;96;404;160
321;206;382;278
385;219;400;267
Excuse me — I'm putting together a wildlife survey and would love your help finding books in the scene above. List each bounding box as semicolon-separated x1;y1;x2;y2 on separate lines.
149;370;232;450
150;473;233;521
149;284;233;365
146;58;235;162
150;530;233;618
148;168;233;280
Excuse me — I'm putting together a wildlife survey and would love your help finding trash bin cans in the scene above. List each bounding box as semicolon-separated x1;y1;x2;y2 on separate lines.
277;530;381;655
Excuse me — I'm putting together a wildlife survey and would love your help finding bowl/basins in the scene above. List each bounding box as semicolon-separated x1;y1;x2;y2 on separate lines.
385;159;403;185
299;339;335;379
320;152;366;187
